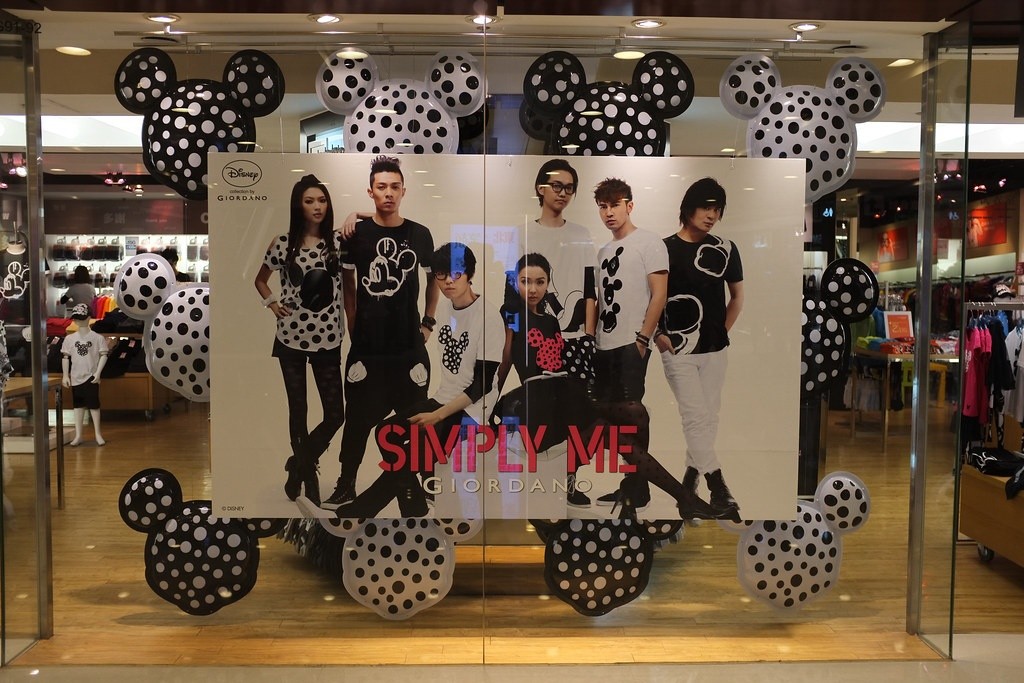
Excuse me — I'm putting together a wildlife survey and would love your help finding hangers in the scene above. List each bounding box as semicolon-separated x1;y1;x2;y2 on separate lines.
847;271;1024;343
845;363;887;388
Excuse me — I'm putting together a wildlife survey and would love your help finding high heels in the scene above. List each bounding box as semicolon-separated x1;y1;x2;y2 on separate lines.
610;478;648;515
283;456;301;502
304;477;320;506
679;503;736;519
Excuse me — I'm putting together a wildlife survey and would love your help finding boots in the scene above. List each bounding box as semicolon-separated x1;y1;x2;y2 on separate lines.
676;465;701;507
704;469;739;510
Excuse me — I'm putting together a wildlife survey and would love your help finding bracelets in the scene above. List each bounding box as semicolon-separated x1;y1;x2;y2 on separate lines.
261;295;278;308
636;338;649;347
634;332;649;343
653;330;663;343
422;316;436;332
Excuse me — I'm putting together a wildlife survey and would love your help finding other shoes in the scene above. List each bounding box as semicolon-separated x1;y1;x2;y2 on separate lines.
597;491;650;507
294;496;338;518
566;474;591;508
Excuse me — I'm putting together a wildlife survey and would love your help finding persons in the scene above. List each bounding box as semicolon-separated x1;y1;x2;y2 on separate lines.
0;242;50;325
254;155;745;520
16;327;32;416
61;303;109;445
60;265;95;318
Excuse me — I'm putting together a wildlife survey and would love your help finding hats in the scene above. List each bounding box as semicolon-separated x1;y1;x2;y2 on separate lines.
70;303;92;319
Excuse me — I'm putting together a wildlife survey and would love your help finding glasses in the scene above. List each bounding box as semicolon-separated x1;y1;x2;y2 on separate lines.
434;269;463;281
541;182;578;197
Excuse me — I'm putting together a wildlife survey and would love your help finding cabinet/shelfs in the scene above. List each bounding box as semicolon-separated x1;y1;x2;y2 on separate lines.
959;464;1024;570
47;333;181;422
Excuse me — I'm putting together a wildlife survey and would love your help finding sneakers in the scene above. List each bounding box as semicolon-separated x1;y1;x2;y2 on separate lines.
322;478;356;508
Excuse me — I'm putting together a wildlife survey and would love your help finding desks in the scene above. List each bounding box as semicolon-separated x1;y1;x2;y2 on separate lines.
850;346;960;455
2;377;65;510
